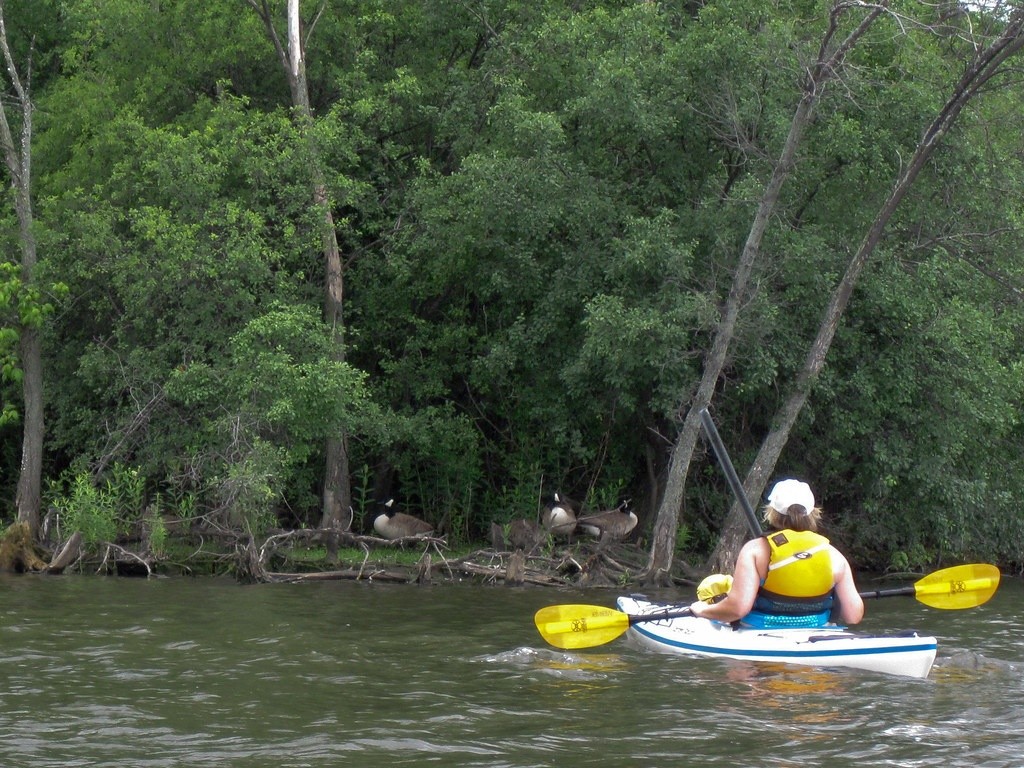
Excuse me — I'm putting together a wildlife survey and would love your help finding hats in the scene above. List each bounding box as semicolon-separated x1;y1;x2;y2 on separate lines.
767;480;815;517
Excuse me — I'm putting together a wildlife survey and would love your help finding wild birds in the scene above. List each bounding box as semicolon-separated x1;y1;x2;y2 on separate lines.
373;498;434;550
541;488;578;544
577;496;640;543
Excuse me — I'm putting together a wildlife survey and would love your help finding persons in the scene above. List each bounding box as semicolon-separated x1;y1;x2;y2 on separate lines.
689;478;863;633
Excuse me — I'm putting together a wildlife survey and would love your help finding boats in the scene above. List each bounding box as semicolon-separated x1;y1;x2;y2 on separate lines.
613;591;938;679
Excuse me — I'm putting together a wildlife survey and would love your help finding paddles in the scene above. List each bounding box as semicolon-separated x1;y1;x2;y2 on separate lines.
534;560;1000;651
